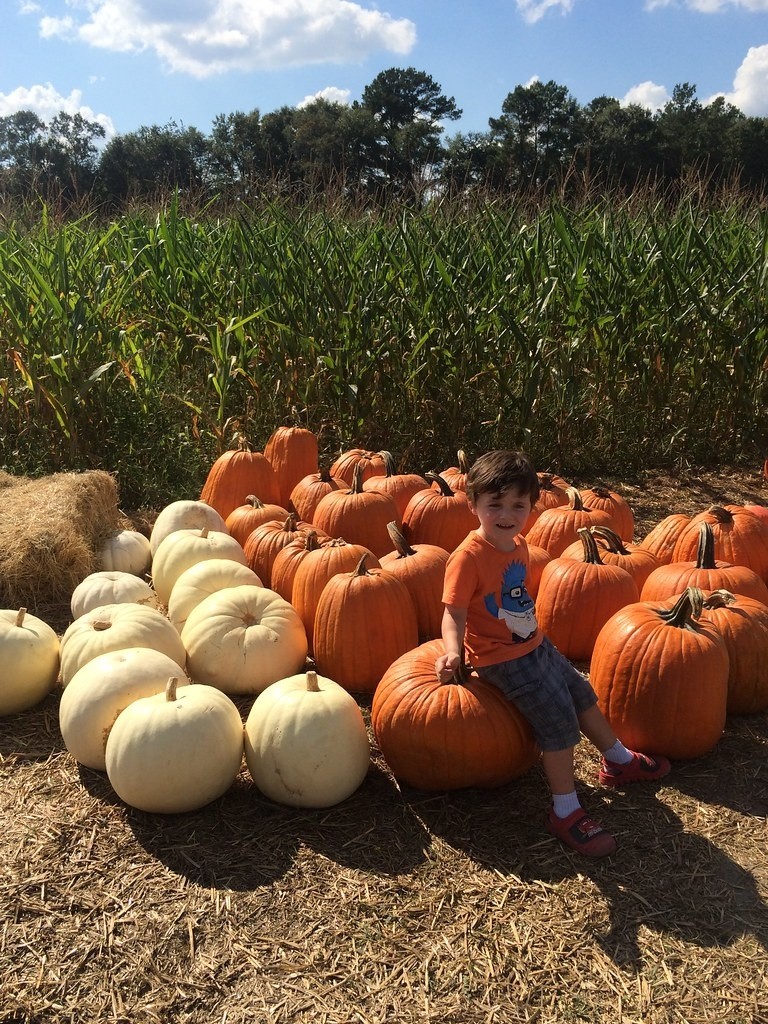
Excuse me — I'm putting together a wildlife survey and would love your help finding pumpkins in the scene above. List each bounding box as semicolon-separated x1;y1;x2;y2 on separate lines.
0;427;768;813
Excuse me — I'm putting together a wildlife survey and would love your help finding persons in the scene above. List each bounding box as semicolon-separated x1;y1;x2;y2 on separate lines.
436;450;671;854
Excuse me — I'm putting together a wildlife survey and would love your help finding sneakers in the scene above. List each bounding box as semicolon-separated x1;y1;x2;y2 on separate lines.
545;801;617;858
599;751;672;786
